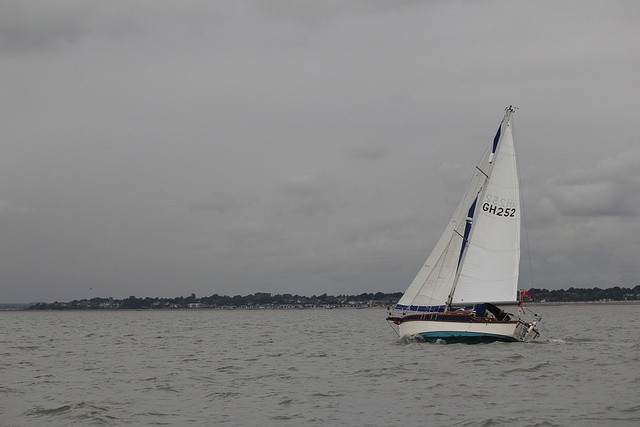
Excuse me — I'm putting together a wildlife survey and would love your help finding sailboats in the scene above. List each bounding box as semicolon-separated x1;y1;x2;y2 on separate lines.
384;104;542;343
